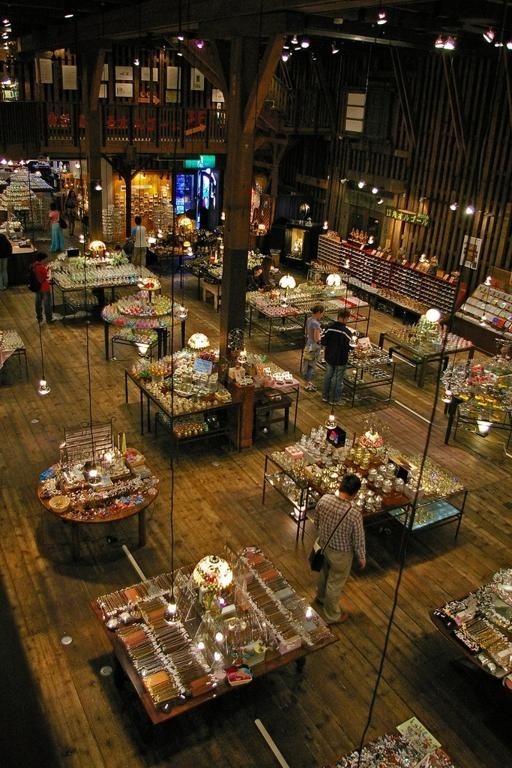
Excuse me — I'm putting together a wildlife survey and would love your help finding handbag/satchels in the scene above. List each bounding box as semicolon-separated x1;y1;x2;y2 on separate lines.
59;211;68;230
308;536;325;573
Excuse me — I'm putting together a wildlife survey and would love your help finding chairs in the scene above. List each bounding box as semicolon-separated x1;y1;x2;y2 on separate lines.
48;108;207;142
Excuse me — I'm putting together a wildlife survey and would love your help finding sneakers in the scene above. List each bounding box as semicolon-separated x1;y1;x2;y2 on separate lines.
38;317;57;325
321;397;348;408
0;285;9;292
325;612;350;626
304;382;320;392
314;596;324;609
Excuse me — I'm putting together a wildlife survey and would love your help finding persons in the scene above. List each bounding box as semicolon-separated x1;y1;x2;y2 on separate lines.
1;233;13;291
29;252;57;323
311;473;366;625
130;216;148;268
301;302;321;391
64;190;77;237
48;203;65;253
319;309;351;406
244;265;267;294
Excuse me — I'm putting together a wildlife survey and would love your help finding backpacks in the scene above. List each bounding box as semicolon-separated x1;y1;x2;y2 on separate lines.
26;263;47;293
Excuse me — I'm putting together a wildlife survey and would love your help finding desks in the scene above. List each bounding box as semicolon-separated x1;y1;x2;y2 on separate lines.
333;717;456;768
427;565;512;695
244;269;374;355
0;221;38;288
122;347;302;465
93;545;342;750
45;240;157;325
37;451;159;559
300;333;398;410
102;278;189;362
148;227;278;313
1;329;30;380
377;323;476;390
263;415;469;561
441;354;511;452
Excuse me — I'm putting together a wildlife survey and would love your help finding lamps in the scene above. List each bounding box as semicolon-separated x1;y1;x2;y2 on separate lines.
280;0;497;63
